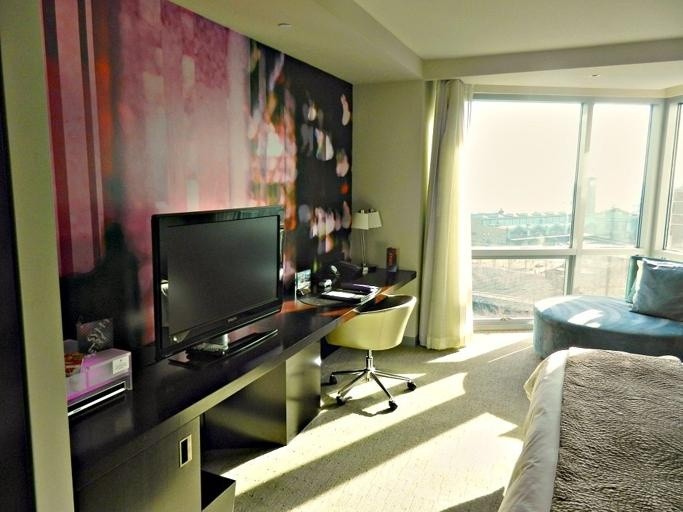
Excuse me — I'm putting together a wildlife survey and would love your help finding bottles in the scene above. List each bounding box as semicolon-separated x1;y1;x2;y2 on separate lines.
386;248;396;272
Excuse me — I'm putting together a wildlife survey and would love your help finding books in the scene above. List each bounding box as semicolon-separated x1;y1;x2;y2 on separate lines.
320;284;378;303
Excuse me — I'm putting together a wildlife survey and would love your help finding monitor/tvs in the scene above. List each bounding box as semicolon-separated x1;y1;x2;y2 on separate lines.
151;204;285;370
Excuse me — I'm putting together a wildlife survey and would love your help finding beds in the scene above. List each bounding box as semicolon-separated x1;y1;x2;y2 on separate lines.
498;346;683;511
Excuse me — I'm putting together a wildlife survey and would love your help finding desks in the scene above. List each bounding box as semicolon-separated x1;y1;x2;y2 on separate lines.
71;268;417;512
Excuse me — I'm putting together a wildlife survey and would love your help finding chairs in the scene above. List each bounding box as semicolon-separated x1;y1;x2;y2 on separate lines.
325;294;417;410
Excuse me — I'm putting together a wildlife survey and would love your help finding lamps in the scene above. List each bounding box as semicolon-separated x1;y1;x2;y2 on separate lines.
351;209;382;275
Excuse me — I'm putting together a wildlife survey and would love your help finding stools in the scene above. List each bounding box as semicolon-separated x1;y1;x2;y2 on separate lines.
534;253;683;361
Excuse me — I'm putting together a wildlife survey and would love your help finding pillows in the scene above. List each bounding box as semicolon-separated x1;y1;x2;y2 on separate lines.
630;259;683;321
